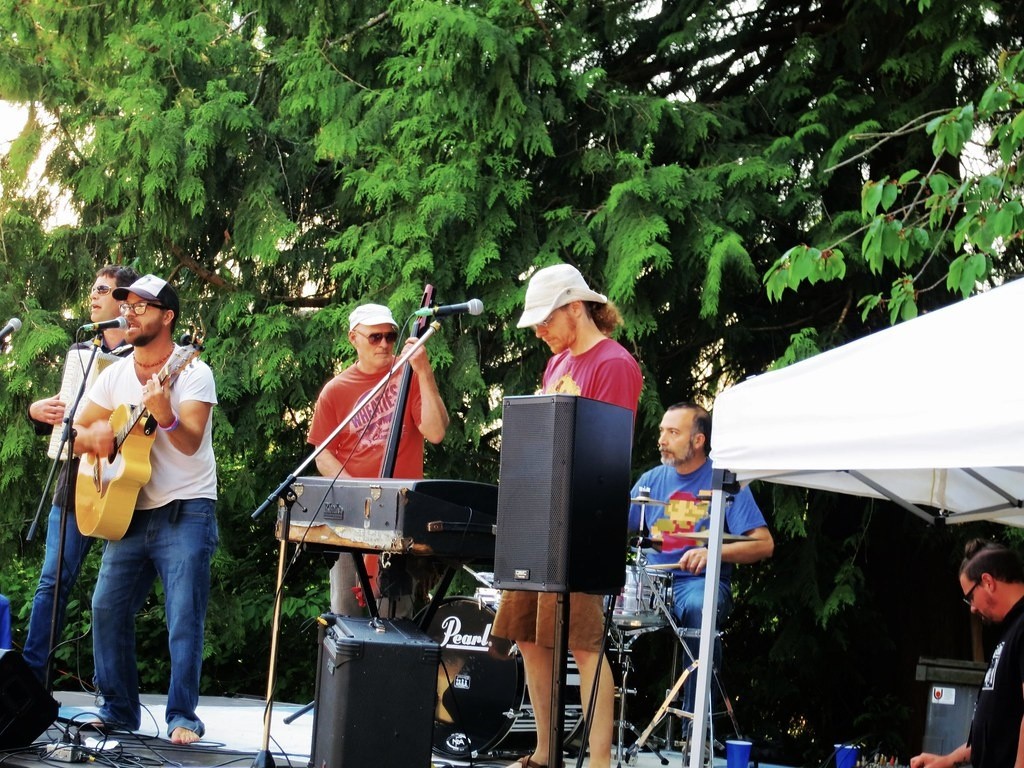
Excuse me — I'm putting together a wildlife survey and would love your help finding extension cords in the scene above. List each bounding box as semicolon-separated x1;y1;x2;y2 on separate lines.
47;742;89;763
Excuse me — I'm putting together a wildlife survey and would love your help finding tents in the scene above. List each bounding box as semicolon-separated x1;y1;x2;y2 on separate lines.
689;274;1024;768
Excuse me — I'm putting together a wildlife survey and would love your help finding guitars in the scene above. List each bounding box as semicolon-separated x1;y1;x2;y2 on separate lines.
75;316;207;543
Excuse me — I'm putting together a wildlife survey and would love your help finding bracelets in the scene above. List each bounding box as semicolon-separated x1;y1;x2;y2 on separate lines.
157;416;179;433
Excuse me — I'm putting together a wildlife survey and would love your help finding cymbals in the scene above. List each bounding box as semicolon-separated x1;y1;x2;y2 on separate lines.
630;496;669;506
668;527;762;545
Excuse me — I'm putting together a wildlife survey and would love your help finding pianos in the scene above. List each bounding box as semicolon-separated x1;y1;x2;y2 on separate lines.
271;474;497;631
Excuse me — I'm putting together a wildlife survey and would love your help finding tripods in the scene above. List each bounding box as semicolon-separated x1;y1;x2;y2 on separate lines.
614;503;759;768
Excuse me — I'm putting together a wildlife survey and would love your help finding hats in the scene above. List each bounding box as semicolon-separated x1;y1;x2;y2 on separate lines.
113;275;179;315
517;264;607;327
349;304;400;330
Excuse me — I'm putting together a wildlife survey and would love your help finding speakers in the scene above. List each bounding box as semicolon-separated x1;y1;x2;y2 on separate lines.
0;648;59;754
309;612;440;768
494;396;634;594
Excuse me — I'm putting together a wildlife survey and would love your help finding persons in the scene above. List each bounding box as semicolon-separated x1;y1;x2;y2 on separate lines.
25;268;137;688
909;536;1024;768
626;402;773;751
490;264;643;768
307;304;450;619
70;274;220;743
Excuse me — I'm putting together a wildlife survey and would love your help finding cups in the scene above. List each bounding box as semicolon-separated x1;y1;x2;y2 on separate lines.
726;740;752;768
834;744;860;768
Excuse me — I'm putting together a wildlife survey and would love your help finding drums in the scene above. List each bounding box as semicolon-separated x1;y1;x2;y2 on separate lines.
411;596;584;763
603;565;674;628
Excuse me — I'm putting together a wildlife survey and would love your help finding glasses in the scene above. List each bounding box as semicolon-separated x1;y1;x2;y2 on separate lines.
964;578;985;606
540;308;564;326
353;330;400;346
120;301;168;315
92;286;115;296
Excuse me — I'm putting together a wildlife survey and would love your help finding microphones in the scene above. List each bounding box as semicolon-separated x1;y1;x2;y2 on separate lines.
416;299;484;316
80;316;127;331
0;318;22;340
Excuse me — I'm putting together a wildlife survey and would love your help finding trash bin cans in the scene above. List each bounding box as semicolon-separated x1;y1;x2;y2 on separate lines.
915;658;990;757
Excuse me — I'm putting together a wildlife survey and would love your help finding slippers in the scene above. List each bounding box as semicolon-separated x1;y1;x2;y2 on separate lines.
508;755;566;768
